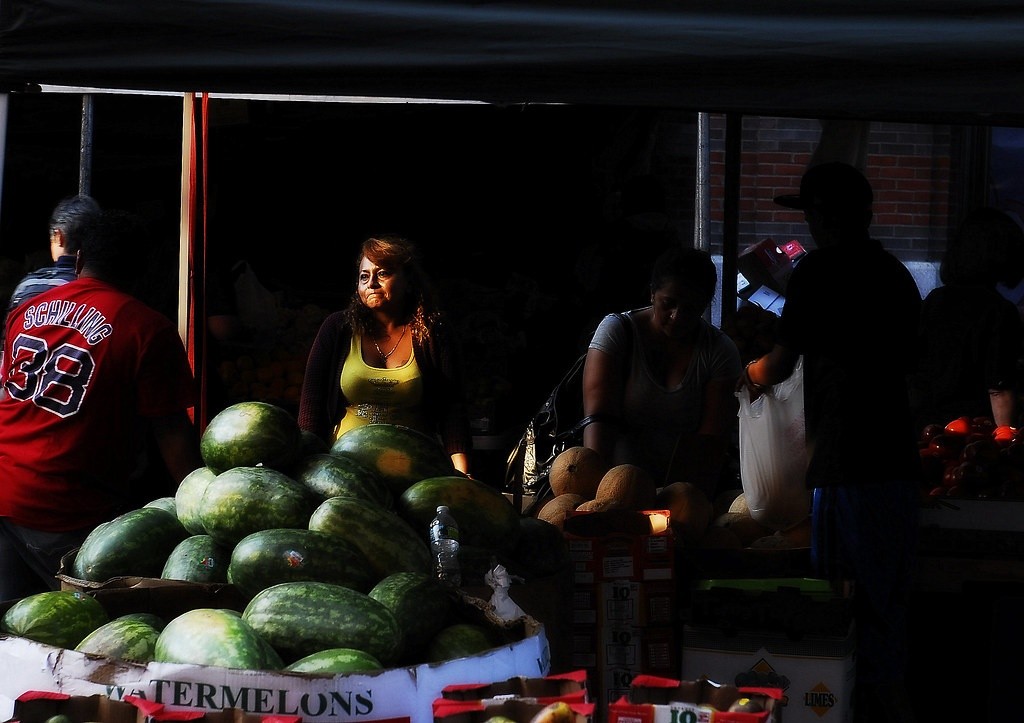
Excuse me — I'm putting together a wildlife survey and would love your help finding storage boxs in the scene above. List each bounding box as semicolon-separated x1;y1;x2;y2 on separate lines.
0;586;866;723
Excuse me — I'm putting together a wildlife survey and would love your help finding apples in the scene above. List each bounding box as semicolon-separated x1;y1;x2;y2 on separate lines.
919;416;1024;498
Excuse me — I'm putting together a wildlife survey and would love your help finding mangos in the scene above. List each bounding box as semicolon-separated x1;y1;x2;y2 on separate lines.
480;698;574;723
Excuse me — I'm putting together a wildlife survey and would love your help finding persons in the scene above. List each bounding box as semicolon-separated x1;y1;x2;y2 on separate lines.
582;246;744;502
7;193;101;314
297;233;473;479
744;160;1024;723
0;212;202;603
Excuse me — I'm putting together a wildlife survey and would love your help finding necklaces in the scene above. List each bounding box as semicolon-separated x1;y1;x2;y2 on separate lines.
372;325;407;363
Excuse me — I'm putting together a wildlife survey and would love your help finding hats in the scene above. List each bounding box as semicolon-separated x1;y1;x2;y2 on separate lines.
774;160;872;213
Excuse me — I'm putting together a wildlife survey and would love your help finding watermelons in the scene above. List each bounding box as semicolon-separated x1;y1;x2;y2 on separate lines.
0;401;519;675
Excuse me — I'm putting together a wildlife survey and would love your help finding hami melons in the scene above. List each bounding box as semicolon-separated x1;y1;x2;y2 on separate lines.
536;446;810;551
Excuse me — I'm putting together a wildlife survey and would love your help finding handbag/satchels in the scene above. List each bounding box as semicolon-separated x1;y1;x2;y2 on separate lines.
737;354;811;524
503;394;577;517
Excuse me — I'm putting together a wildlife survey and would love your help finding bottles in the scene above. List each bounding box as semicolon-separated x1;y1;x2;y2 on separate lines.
428;506;462;590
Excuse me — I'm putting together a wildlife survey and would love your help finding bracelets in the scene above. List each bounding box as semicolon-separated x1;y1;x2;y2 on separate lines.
745;362;764;388
